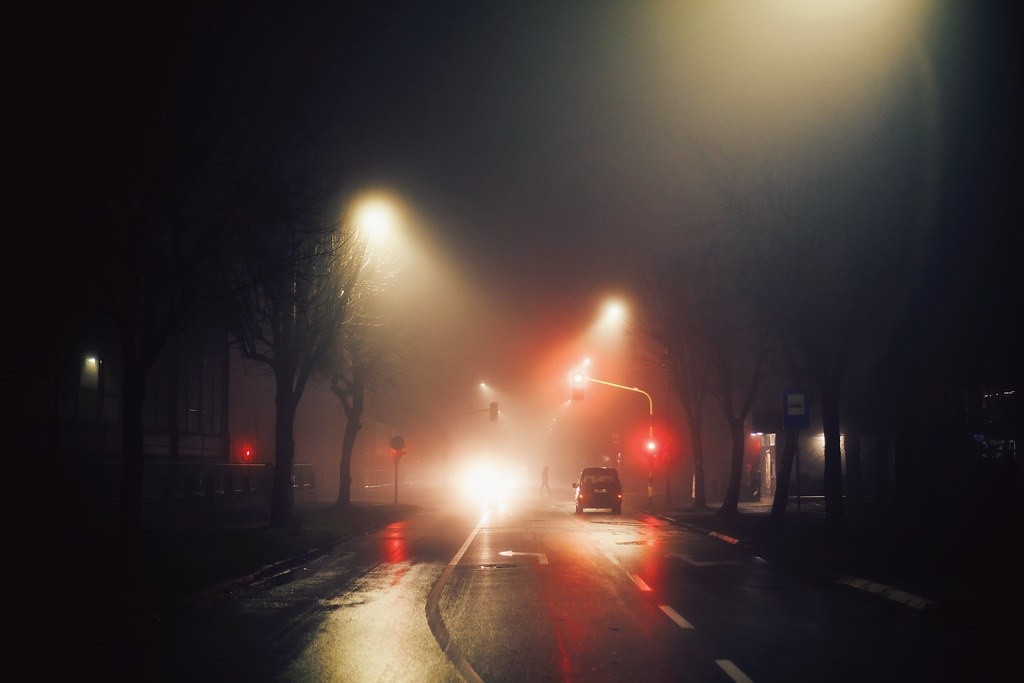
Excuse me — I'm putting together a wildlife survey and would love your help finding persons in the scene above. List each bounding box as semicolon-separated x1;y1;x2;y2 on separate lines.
539;466;553;495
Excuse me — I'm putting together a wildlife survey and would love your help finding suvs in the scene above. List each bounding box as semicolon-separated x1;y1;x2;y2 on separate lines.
572;467;623;514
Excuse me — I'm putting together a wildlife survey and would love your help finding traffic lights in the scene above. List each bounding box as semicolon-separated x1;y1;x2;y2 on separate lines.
644;437;659;454
572;371;586;402
